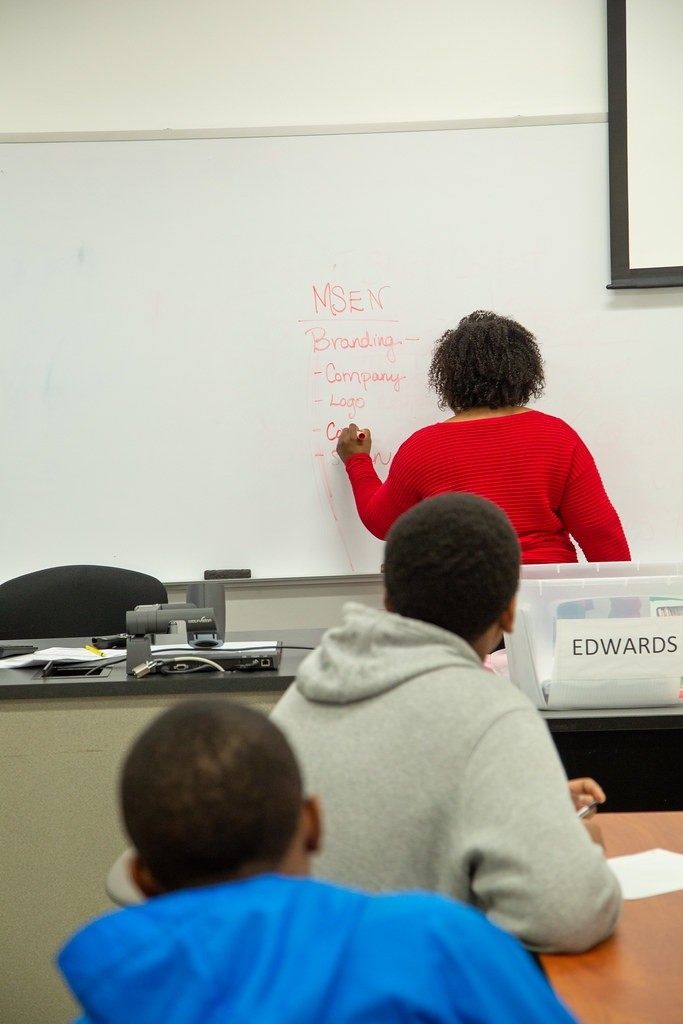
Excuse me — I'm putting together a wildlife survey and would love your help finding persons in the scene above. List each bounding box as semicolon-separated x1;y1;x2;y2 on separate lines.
49;698;578;1024
264;495;623;953
336;309;644;618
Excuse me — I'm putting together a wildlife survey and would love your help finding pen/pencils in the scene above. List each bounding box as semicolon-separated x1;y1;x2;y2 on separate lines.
576;800;600;820
84;644;106;658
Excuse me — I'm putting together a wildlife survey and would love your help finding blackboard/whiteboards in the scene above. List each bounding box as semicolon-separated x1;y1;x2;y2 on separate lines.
0;110;683;590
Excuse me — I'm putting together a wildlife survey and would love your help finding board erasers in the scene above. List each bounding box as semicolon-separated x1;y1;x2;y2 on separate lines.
204;569;252;580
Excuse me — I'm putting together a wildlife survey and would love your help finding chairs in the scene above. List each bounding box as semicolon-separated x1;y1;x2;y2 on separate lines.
0;565;169;640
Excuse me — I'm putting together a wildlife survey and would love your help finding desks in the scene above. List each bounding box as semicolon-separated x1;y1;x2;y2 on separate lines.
535;812;683;1024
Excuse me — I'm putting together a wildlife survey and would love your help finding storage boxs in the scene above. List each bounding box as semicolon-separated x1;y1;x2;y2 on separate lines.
502;562;683;712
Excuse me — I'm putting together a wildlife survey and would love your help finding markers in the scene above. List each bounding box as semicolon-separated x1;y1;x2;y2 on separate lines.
356;430;366;443
